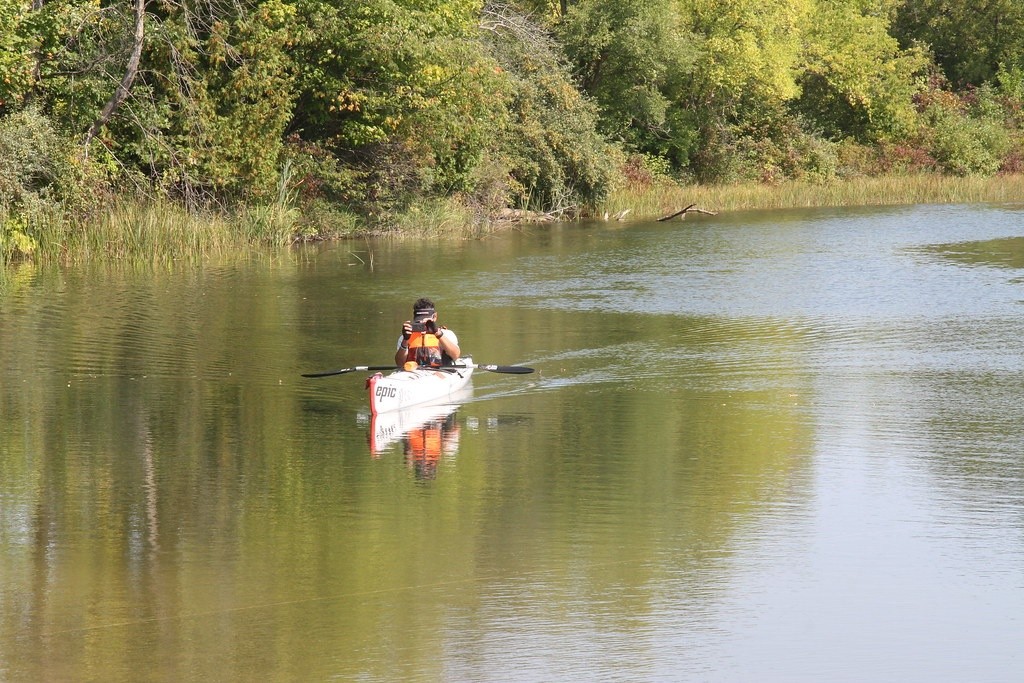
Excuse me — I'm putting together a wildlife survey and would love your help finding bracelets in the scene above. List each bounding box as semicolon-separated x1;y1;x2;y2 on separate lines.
400;343;408;350
436;331;443;339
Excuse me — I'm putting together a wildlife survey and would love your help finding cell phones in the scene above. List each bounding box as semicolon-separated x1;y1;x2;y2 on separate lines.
411;323;424;332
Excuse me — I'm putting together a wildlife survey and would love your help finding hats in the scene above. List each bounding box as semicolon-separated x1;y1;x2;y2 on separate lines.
414;308;435;322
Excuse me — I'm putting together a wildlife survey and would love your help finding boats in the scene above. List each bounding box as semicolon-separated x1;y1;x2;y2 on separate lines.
367;355;475;415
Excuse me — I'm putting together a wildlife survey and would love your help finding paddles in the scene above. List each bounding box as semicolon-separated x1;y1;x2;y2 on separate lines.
301;365;535;378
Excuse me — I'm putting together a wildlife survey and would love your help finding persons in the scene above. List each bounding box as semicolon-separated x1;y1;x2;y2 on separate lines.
395;298;461;368
